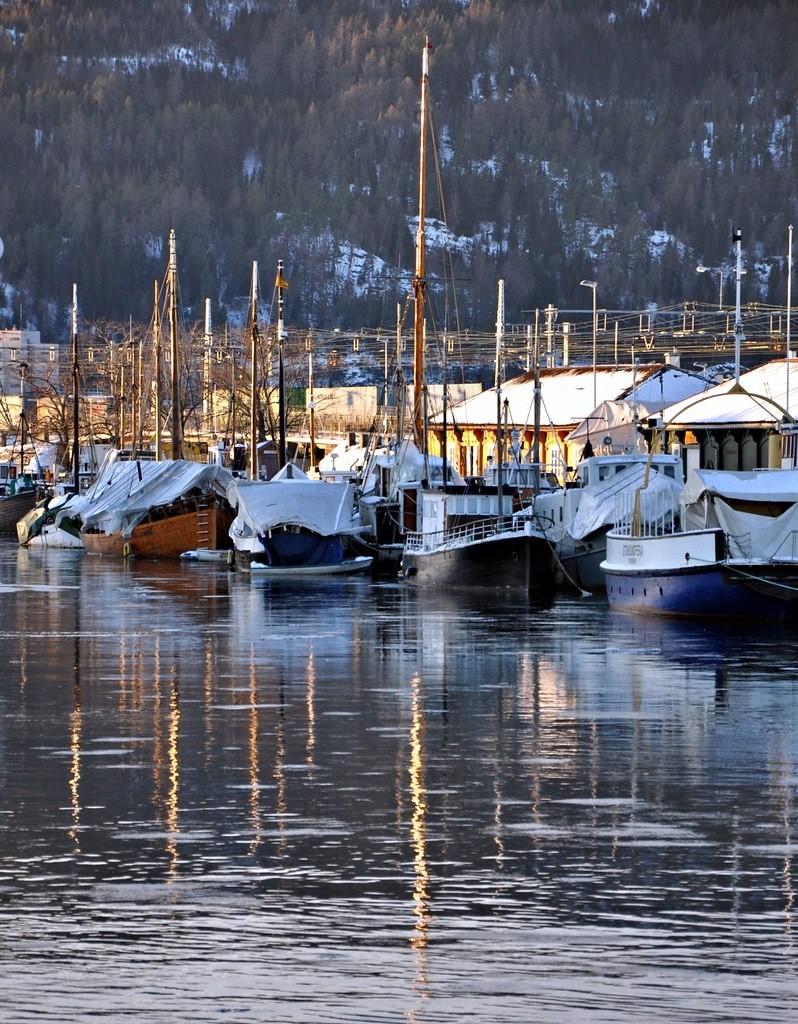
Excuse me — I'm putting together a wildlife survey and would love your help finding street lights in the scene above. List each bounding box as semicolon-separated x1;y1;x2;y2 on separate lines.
696;266;723;312
580;279;597;410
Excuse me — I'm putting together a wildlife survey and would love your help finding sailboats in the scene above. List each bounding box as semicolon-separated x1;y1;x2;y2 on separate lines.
0;34;798;614
